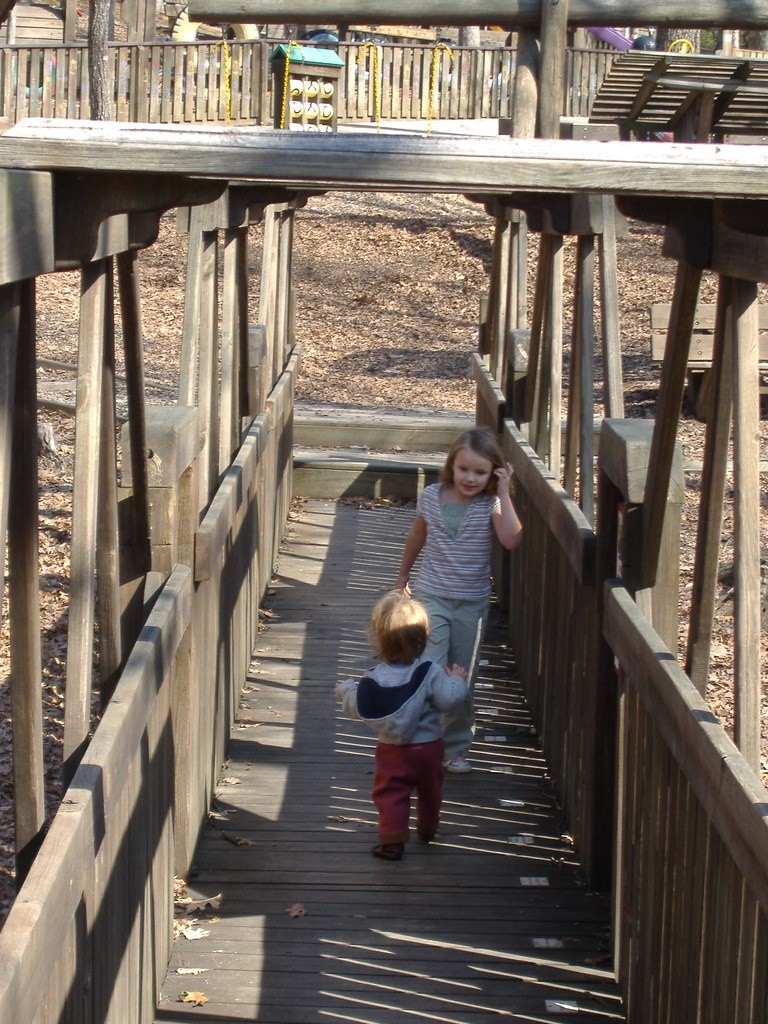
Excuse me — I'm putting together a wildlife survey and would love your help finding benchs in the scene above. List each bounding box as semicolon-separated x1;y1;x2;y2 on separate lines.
589;51;768;143
650;303;768;423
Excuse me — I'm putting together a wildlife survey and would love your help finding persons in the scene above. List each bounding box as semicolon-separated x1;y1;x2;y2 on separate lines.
396;427;523;775
332;587;468;863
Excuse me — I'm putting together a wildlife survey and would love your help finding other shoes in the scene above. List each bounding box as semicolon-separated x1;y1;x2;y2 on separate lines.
443;756;471;772
418;832;434;842
372;843;405;861
